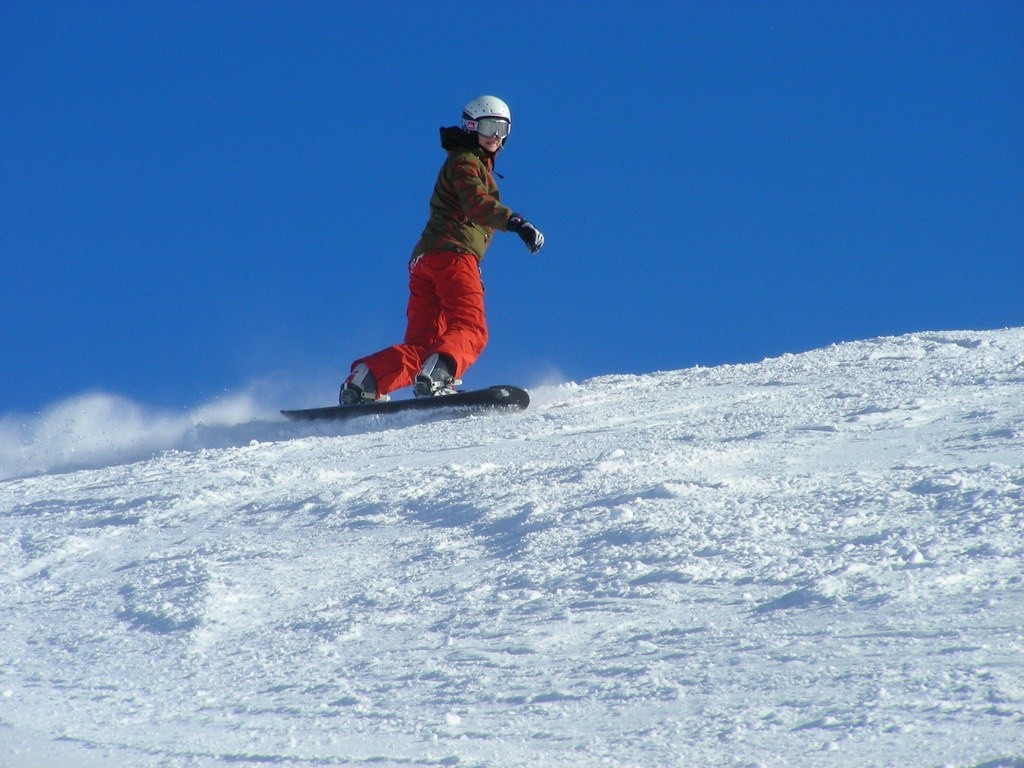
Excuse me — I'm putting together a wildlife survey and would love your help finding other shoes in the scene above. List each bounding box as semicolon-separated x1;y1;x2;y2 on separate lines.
340;383;391;405
414;381;466;397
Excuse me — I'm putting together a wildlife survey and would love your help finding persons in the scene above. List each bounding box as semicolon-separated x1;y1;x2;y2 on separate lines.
341;94;545;402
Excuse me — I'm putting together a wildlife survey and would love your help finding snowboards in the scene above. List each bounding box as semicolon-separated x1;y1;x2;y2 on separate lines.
278;383;531;422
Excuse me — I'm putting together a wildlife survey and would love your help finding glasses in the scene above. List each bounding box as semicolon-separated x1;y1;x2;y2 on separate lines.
467;121;510;138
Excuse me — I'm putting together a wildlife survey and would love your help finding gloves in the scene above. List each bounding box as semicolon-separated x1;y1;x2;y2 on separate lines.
507;213;545;255
478;267;486;292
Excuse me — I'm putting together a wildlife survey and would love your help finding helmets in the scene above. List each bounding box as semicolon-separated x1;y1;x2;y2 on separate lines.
461;96;511;134
